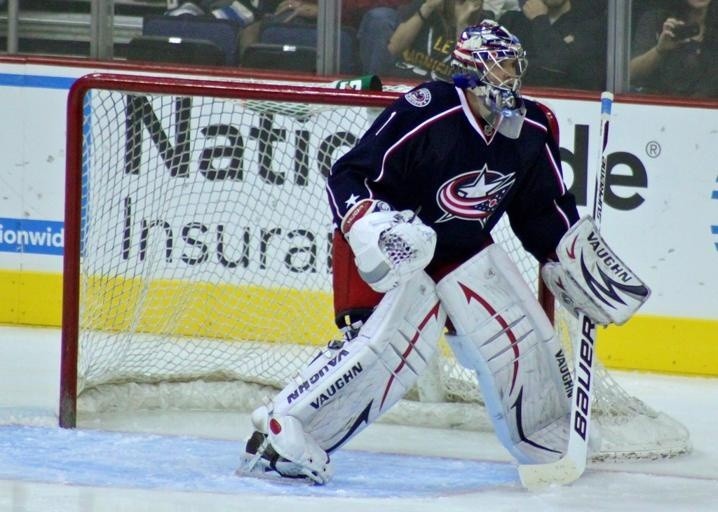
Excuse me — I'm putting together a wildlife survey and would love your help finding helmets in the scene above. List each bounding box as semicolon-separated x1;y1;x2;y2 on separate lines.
449;17;531;90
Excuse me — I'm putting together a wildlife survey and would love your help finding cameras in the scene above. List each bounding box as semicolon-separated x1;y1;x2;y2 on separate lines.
671;22;699;41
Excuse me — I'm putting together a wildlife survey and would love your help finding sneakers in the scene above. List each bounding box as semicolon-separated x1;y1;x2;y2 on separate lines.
244;430;315;479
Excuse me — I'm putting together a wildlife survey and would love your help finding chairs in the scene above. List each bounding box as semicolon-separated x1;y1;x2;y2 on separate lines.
129;15;358;76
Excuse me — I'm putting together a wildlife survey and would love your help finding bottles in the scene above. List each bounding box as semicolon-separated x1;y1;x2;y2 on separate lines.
327;75;382;93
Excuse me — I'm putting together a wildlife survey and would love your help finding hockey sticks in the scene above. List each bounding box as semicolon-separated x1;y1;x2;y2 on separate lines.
516;91;613;492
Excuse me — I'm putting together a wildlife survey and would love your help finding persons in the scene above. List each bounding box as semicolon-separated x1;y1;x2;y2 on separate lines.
159;0;717;99
245;18;580;461
